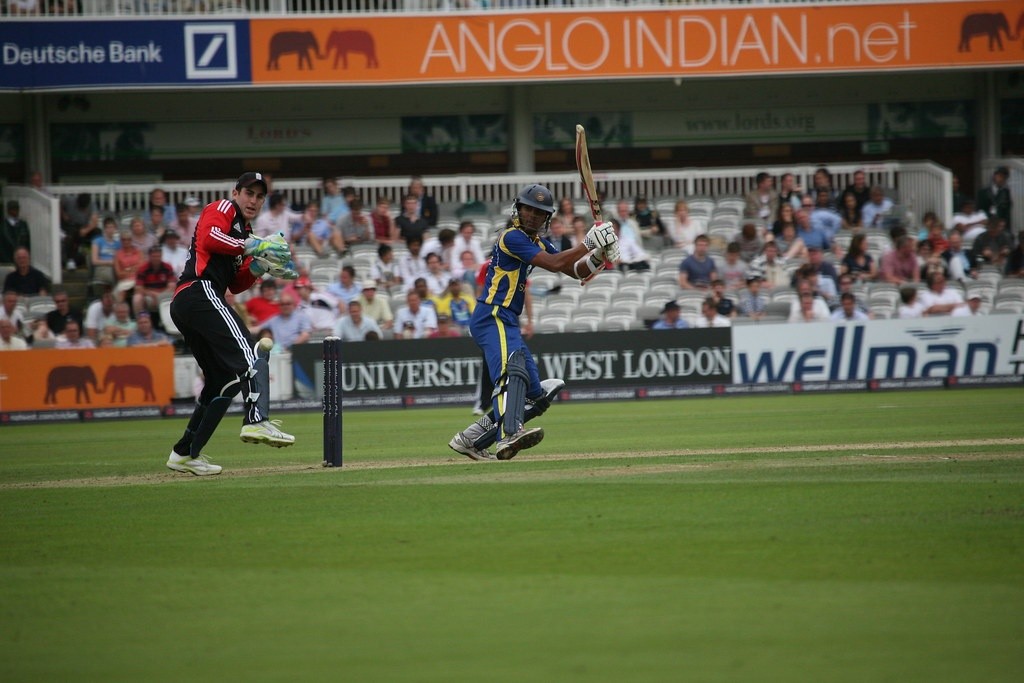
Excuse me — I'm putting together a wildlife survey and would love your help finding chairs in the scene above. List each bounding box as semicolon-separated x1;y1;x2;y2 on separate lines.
0;193;1024;339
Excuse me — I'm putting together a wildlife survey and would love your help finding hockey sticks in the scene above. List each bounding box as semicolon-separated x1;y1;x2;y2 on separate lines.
576;122;612;270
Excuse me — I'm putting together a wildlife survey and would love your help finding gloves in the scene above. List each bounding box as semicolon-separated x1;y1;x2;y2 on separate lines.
585;243;619;272
581;222;617;251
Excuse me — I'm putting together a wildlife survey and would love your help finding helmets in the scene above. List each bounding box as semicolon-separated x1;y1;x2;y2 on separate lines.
514;184;554;214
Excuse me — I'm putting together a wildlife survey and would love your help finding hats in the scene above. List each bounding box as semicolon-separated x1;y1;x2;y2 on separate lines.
160;229;180;243
361;279;378;290
659;303;679;315
183;198;200;206
236;170;267;197
293;277;311;288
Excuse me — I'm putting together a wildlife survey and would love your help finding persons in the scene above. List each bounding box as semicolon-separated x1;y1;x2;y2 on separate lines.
0;172;204;354
474;259;533;416
448;184;620;461
545;165;1024;329
166;173;295;477
226;177;484;343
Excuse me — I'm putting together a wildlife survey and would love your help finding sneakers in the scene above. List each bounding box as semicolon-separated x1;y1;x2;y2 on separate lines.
165;450;222;476
448;431;499;460
240;419;295;448
496;426;544;460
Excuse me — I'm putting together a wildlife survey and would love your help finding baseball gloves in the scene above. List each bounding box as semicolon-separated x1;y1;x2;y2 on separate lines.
248;233;301;281
